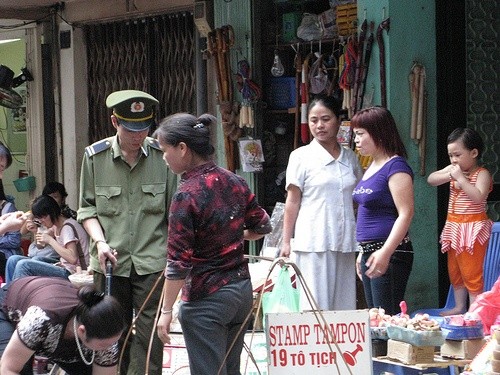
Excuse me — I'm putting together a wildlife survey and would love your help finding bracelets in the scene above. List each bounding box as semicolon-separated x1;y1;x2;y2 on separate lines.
93;240;106;246
161;309;172;315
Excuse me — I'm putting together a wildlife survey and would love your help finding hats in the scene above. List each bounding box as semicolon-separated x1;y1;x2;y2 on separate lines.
106;90;159;132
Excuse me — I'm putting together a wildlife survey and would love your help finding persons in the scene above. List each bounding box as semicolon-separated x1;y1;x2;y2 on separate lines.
5;194;90;283
156;112;272;375
0;142;27;237
77;88;182;374
0;192;23;279
278;99;363;311
0;276;121;374
352;106;416;315
426;128;493;319
18;180;87;233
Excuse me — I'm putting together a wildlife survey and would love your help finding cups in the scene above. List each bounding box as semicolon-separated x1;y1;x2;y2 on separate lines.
36;227;48;247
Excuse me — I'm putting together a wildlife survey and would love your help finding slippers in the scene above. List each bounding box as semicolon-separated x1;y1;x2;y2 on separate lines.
439;310;464;316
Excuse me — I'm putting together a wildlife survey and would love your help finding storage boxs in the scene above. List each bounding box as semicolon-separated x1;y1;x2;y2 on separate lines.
266;78;295;108
370;324;484;365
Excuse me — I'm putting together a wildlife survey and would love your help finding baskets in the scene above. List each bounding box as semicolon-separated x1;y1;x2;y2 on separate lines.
261;77;296;108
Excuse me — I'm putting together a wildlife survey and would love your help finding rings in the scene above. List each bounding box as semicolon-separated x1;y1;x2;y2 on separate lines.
376;270;383;274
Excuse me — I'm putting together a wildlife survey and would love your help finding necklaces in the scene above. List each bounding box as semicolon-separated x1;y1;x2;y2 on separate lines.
73;316;96;365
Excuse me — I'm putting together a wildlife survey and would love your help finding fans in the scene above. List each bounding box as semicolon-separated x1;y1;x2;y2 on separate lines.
0;64;34;110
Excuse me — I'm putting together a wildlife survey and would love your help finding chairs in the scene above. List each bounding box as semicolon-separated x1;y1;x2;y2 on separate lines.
411;222;500;318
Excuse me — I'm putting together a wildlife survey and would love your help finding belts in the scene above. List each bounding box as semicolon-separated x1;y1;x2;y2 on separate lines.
357;236;410;254
